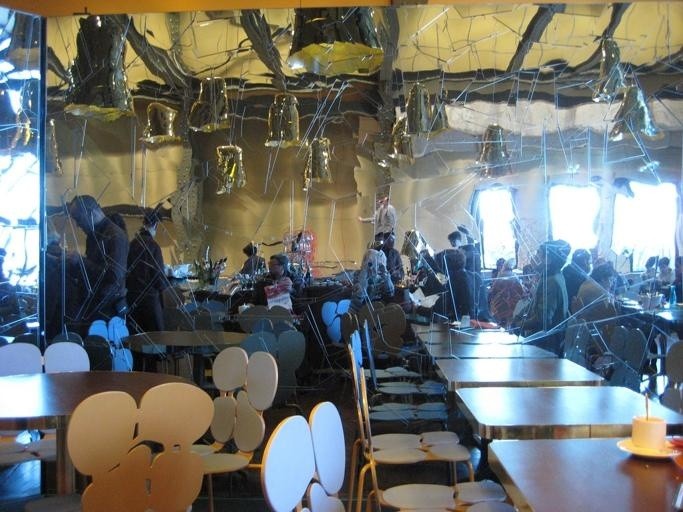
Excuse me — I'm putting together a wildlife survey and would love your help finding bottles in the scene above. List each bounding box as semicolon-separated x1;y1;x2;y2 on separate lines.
668;285;676;307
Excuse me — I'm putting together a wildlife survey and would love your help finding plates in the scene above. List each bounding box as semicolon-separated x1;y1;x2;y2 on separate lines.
617;440;679;460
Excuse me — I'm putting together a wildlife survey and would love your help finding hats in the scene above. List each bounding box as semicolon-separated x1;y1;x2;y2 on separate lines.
539;241;571;264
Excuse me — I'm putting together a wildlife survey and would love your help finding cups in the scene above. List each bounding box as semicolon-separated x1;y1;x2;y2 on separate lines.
631;416;666;451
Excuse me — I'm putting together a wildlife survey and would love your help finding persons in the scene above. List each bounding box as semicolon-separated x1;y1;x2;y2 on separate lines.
241;243;266;276
0;195;181;372
251;255;300;313
356;195;395;249
347;226;682;374
